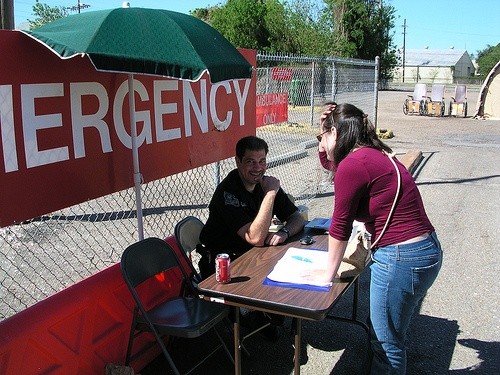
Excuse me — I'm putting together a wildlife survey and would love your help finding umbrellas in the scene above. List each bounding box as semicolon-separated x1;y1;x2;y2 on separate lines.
12;2;252;241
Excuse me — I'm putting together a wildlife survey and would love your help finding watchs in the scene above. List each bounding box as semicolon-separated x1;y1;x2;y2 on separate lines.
278;226;290;239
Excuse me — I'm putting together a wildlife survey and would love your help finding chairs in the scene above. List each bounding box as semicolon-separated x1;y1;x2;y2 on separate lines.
403;84;468;118
120;216;281;375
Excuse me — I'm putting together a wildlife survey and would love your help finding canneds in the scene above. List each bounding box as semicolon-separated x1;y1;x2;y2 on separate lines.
215;253;231;284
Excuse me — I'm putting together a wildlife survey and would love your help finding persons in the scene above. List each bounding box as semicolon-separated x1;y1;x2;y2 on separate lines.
198;136;305;338
302;103;443;375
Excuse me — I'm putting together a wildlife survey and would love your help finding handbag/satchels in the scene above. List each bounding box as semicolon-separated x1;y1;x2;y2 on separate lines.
337;230;369;278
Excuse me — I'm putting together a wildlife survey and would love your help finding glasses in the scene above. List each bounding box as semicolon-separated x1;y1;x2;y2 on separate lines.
317;129;331;142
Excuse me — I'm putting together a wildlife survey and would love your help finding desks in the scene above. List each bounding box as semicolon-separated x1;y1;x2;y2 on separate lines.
197;222;373;375
